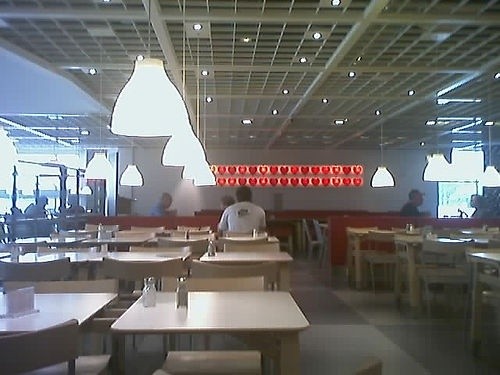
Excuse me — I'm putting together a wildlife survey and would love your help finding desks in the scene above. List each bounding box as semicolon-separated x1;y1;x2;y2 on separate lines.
0;230;500;375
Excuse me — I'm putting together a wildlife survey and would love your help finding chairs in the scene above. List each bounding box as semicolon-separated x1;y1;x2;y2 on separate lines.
301;217;500;348
0;223;269;375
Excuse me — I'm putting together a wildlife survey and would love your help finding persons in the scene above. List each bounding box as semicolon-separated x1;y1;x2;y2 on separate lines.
150;190;175;216
216;184;267;234
401;189;423;216
470;193;496;218
23;196;49;221
61;194;86;231
219;197;236;210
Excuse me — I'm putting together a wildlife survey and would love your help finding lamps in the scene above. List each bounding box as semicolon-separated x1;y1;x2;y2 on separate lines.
83;0;217;188
369;73;500;188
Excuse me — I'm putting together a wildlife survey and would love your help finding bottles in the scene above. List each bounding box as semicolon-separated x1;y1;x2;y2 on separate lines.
142;277;157;307
0;287;6;316
208;240;216;257
184;229;189;239
207;230;216;241
175;276;188;309
253;228;257;238
